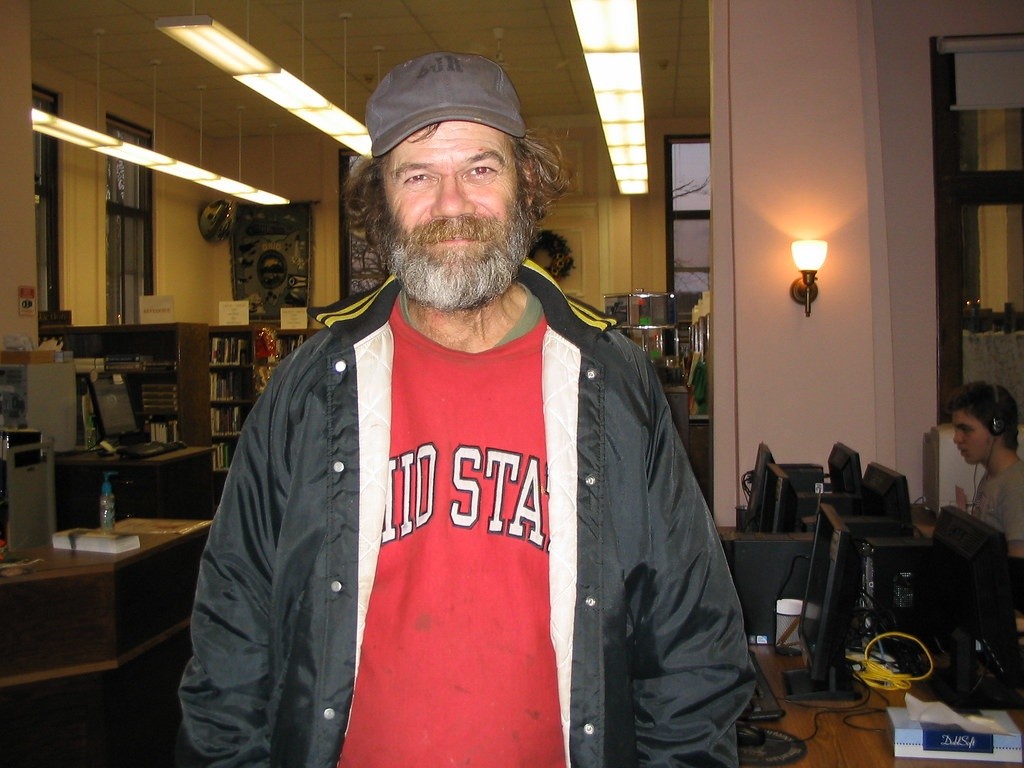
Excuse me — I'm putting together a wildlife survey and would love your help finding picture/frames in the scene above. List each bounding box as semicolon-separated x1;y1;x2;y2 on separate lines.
228;201;314;324
338;147;389;300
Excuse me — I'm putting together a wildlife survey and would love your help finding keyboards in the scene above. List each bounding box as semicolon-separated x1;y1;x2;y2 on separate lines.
117;441;180;458
735;650;786;722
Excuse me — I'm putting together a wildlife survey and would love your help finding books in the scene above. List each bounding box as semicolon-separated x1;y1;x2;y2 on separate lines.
52;527;140;553
210;334;303;470
73;352;178;443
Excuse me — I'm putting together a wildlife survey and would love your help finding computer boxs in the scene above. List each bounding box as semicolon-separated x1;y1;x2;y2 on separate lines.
722;462;940;656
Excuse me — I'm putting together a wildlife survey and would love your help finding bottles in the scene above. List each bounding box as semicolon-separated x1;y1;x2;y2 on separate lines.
775;598;803;649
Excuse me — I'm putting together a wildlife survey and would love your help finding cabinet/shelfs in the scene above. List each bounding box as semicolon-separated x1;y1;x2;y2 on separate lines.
208;324;275;508
39;322;215;531
274;329;319;363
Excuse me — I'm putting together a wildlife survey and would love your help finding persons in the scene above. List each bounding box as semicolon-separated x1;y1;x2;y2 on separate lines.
177;55;758;767
947;380;1024;602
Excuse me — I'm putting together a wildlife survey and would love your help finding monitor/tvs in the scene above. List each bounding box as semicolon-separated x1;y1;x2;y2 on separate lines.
83;370;143;456
742;441;1024;711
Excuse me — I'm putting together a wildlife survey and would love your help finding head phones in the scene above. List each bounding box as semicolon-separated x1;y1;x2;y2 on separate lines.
986;382;1006;435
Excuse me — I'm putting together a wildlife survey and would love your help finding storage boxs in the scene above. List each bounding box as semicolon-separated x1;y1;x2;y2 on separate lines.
138;295;174;324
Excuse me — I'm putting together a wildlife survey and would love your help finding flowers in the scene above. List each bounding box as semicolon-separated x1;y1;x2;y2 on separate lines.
528;229;576;282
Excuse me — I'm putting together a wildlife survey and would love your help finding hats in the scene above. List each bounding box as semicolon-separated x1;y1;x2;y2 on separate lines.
365;52;526;159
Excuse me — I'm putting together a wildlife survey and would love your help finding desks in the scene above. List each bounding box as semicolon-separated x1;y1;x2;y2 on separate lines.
50;443;217;518
736;641;1024;768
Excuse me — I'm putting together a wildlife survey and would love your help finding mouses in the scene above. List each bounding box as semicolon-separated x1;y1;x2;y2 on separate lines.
735;721;766;745
170;441;188;449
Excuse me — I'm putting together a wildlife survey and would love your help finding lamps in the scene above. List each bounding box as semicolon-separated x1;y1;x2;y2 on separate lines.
790;240;828;319
567;0;651;196
33;0;381;206
